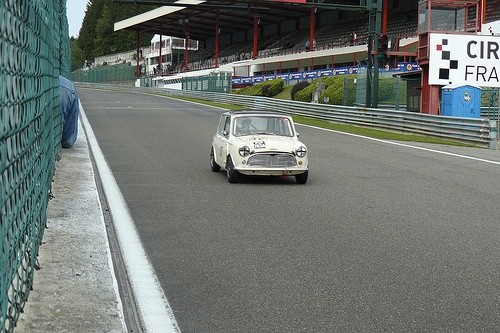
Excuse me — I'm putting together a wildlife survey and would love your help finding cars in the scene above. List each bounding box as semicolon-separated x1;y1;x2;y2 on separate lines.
208;107;309;187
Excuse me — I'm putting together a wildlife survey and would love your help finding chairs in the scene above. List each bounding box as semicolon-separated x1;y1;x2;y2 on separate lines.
172;1;500;66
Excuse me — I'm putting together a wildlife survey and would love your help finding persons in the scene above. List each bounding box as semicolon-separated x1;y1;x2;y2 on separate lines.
305;39;316;52
